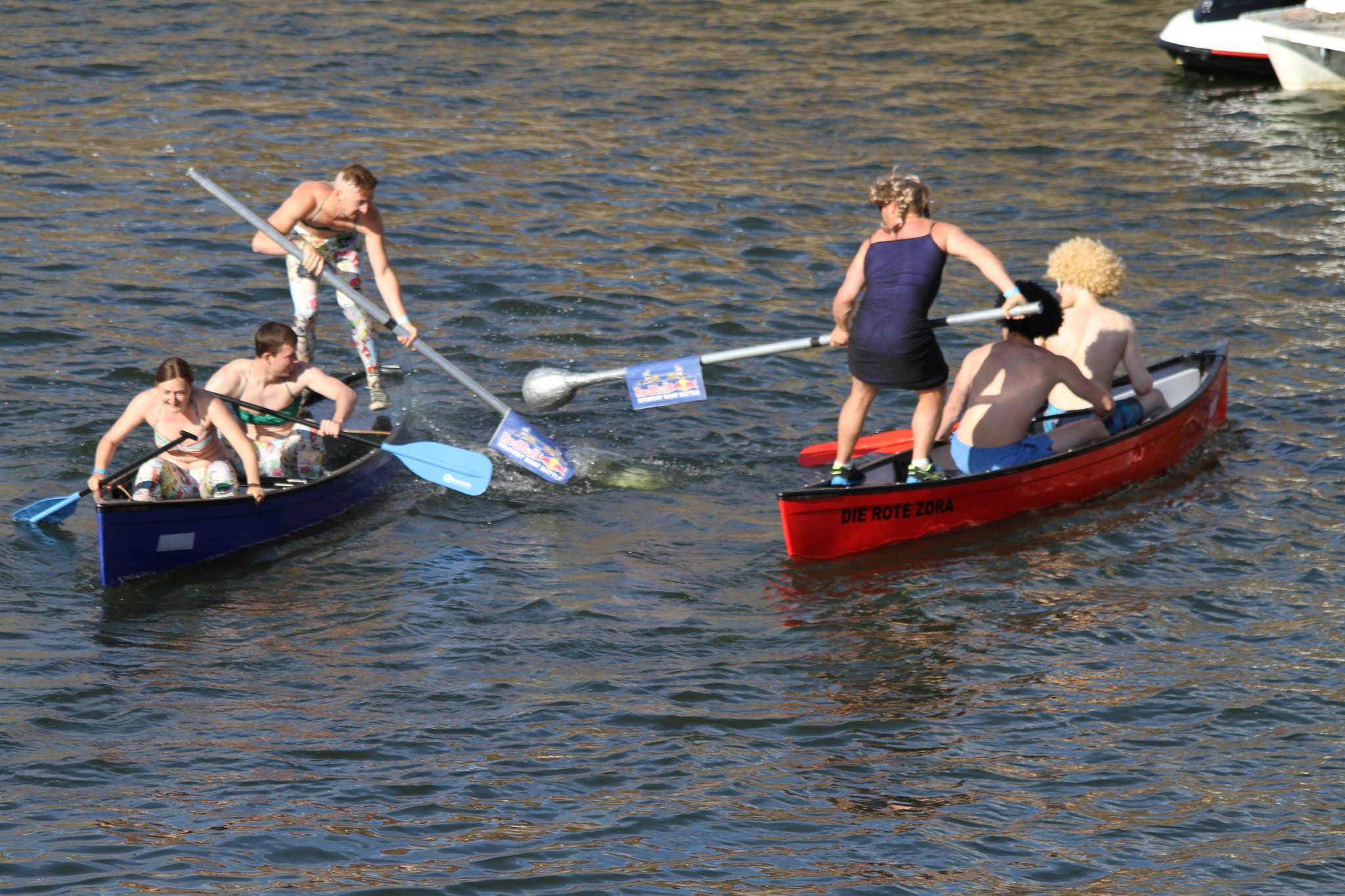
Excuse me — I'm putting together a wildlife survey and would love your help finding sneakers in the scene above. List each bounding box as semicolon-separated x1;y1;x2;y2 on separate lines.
300;387;311;406
906;462;949;484
365;380;391;411
830;465;867;487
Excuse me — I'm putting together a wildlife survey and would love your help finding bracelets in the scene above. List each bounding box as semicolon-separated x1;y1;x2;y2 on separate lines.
395;316;411;327
1003;287;1019;299
93;468;108;477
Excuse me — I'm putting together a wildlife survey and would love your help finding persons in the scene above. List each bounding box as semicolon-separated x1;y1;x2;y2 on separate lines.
251;162;418;412
87;357;266;502
828;172;1027;485
1034;236;1172;436
933;278;1111;474
203;322;358;480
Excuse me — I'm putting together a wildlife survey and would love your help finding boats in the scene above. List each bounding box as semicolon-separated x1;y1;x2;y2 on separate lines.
776;339;1232;561
1155;0;1306;83
96;364;414;585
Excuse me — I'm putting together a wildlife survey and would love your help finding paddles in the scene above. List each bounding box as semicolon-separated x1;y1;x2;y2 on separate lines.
12;430;200;523
796;407;1107;471
194;388;493;499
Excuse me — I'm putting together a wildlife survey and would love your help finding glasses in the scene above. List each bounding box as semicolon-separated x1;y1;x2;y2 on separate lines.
1056;281;1063;287
